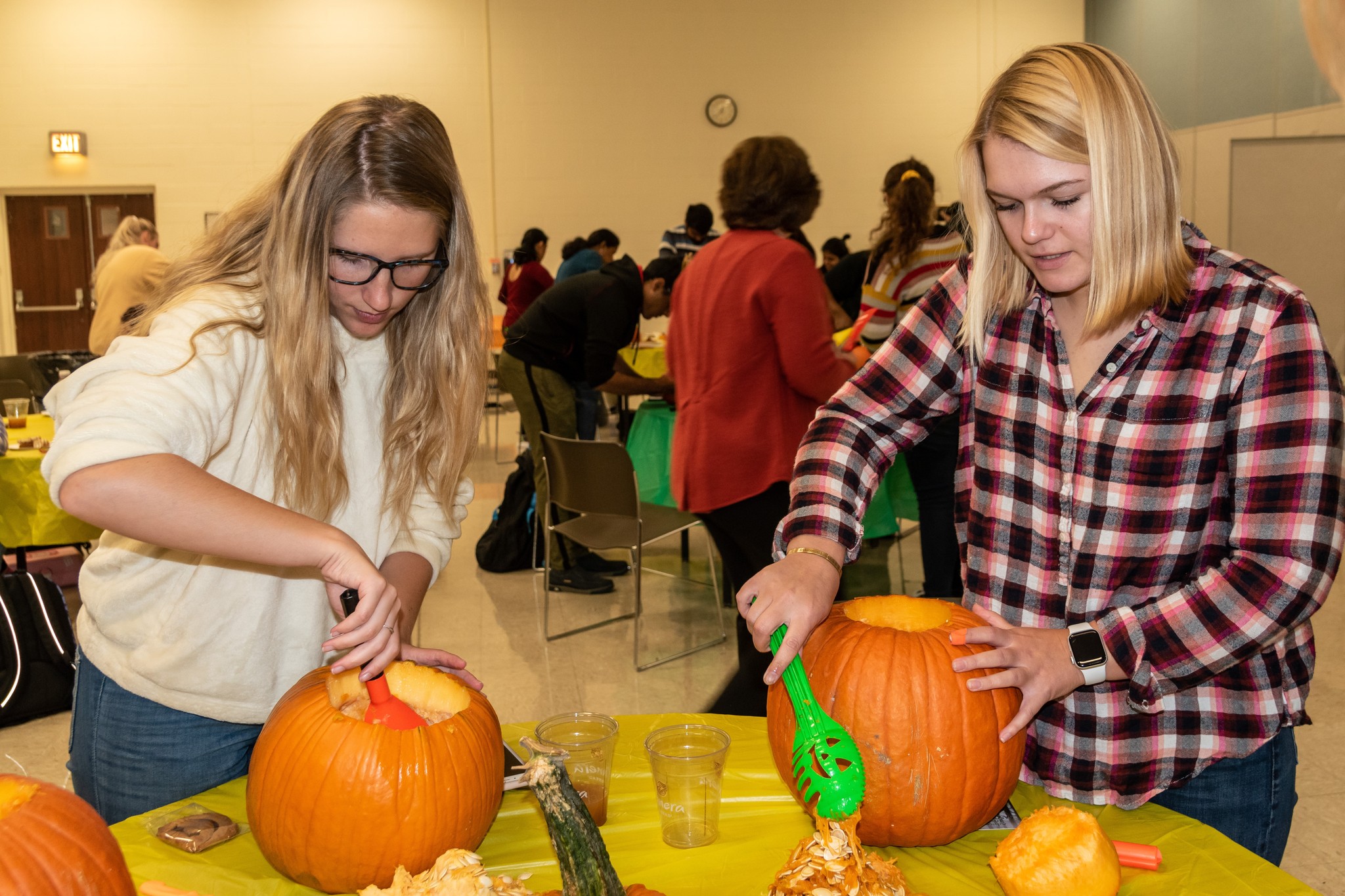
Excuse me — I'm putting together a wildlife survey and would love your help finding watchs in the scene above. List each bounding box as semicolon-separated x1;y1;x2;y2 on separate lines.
1067;621;1108;688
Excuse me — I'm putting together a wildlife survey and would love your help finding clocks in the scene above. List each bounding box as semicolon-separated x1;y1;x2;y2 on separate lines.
705;94;738;128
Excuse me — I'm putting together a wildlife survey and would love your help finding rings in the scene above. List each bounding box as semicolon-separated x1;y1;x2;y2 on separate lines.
384;625;394;635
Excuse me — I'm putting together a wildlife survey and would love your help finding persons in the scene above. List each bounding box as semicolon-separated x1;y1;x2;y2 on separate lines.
858;155;977;602
659;202;725;262
496;247;687;592
663;129;875;715
497;223;560;344
820;248;877;333
37;91;490;826
87;213;180;362
738;47;1344;864
812;231;852;277
553;225;622;440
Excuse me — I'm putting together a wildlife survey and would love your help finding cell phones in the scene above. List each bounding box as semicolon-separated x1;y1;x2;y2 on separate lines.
502;737;529;791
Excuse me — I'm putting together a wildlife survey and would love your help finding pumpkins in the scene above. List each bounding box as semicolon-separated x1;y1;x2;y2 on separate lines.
0;774;139;896
766;593;1026;848
246;659;504;895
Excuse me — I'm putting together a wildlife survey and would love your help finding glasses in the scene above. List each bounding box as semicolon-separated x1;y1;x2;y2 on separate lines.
326;239;449;291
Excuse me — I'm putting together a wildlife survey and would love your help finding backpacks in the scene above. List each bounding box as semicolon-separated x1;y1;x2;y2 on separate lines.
0;546;76;729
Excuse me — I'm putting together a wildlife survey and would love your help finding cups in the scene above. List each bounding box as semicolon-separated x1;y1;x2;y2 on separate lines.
3;397;29;428
647;721;731;849
532;711;620;829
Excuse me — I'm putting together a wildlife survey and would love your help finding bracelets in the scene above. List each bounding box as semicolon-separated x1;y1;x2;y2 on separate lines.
787;549;844;577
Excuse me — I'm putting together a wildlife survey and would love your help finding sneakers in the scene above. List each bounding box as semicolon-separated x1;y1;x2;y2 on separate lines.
548;568;613;595
580;551;629;577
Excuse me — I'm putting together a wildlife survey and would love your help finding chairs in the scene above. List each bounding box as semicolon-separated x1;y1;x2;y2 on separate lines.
535;428;729;672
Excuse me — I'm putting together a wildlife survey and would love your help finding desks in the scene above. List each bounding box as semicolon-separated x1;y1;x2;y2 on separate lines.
624;398;919;543
0;410;104;571
113;713;1318;893
